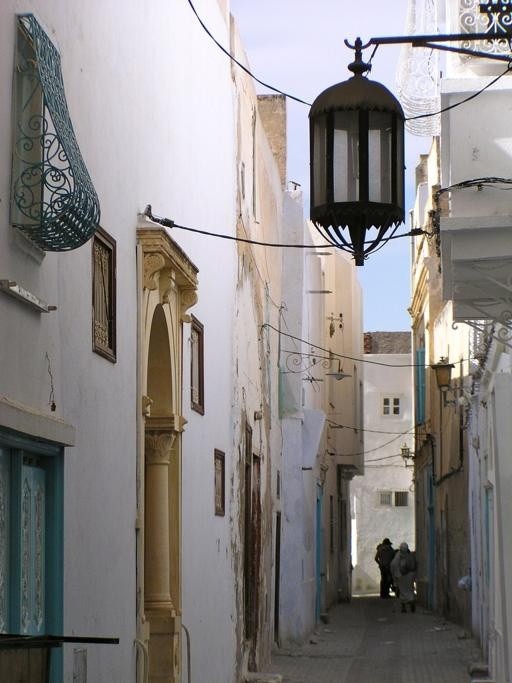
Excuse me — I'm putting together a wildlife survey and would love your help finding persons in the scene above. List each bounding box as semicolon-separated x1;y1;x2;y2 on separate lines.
375;538;395;599
391;543;417;614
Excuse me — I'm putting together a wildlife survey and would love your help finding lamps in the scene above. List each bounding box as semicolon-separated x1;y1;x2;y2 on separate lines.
277;348;354;384
400;442;416;469
433;355;464;406
307;21;511;265
